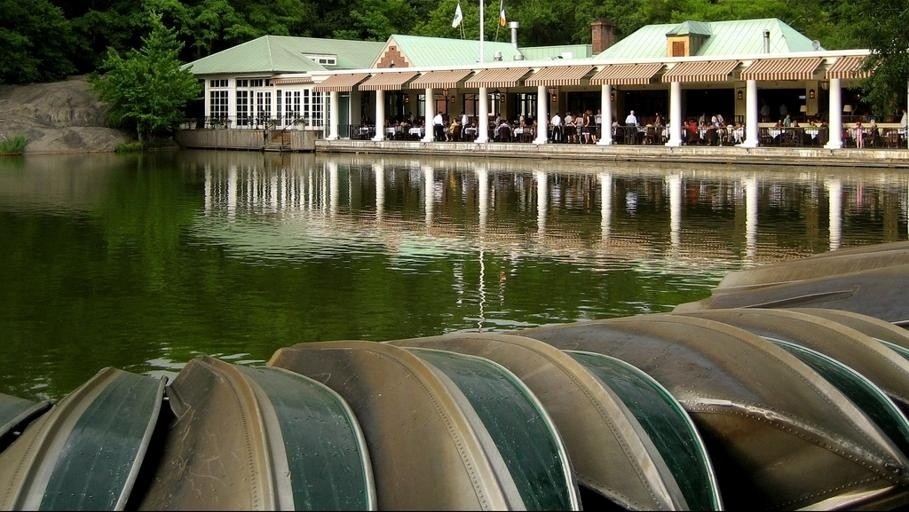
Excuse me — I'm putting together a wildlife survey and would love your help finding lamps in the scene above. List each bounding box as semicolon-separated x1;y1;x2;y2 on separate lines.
405;96;410;104
610;93;614;102
809;88;815;99
451;95;456;104
737;91;743;100
552;95;557;102
501;95;505;103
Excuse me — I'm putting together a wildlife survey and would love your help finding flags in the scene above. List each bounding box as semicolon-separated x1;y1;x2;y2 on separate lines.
500;10;506;27
452;2;462;28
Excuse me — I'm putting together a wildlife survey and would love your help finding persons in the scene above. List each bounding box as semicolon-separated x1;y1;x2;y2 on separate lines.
491;109;602;144
395;110;476;141
774;110;907;148
612;109;746;146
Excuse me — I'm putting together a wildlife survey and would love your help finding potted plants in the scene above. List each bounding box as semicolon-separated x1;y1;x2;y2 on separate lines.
296;118;306;130
